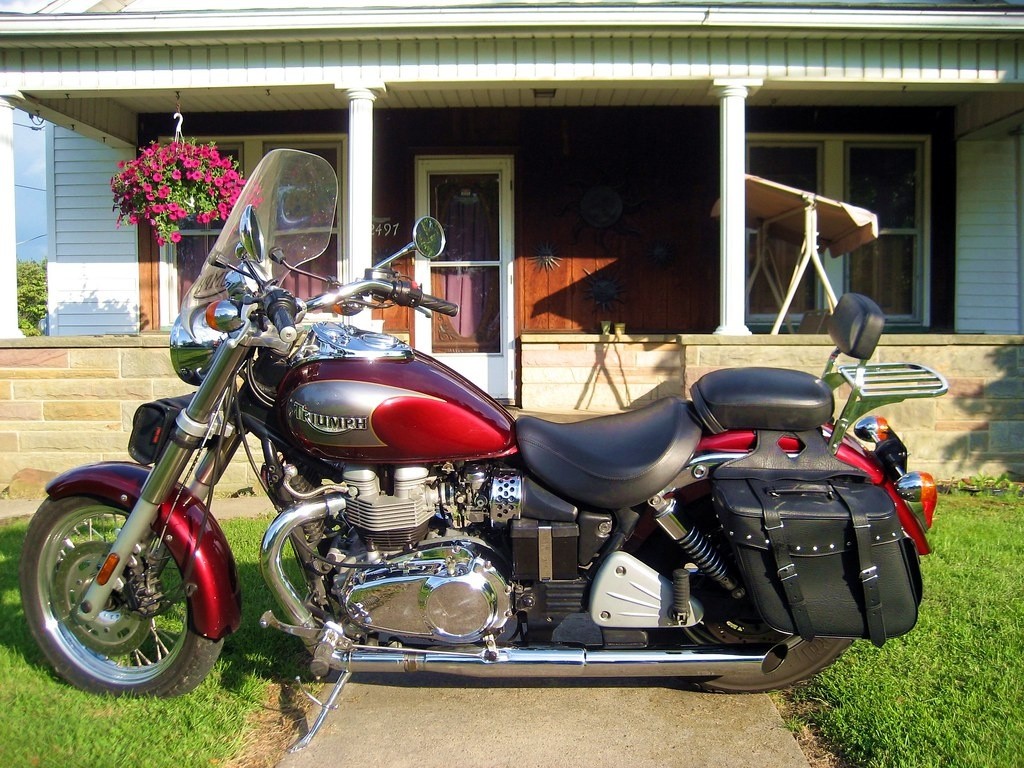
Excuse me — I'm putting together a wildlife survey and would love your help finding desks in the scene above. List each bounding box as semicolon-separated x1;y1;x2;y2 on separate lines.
711;173;879;335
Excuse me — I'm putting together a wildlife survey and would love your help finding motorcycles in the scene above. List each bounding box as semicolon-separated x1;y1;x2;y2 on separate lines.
16;148;948;703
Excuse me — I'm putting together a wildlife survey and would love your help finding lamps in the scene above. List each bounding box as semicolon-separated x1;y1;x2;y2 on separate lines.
532;88;556;98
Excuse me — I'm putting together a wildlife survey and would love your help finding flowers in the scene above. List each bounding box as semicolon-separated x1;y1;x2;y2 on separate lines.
111;136;263;247
